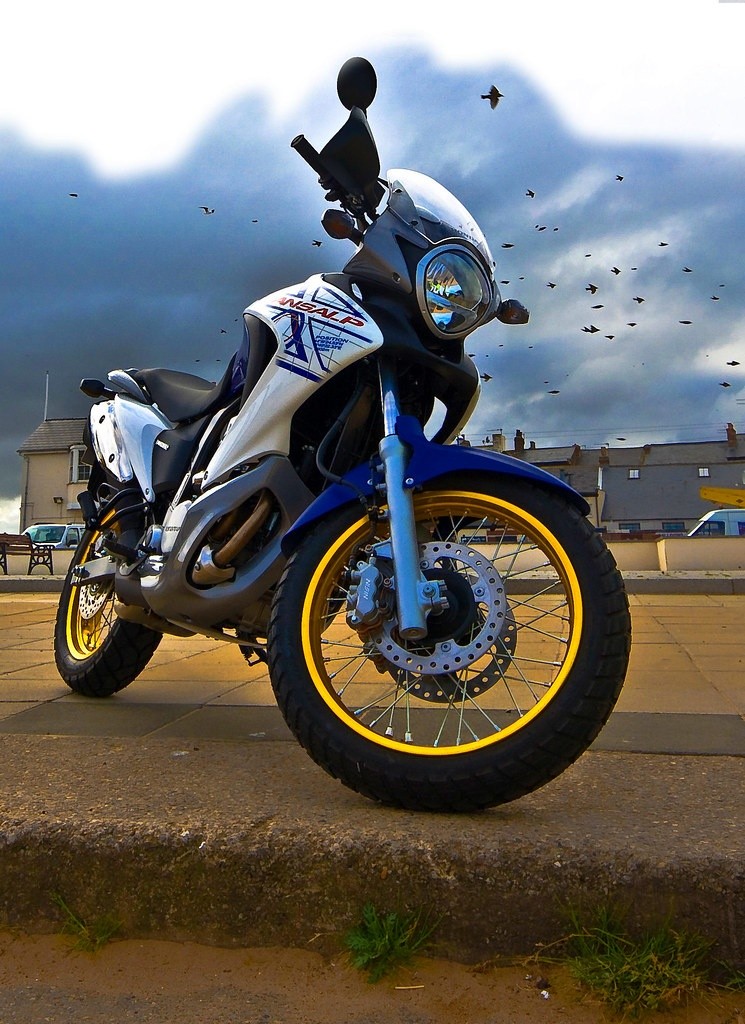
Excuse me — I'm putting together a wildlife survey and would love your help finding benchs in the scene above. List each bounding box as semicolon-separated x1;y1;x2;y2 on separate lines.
0;532;55;575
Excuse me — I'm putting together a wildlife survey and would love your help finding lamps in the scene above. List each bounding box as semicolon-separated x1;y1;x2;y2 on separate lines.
53;497;63;504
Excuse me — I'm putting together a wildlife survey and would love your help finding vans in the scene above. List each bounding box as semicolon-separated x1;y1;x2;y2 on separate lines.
683;509;745;537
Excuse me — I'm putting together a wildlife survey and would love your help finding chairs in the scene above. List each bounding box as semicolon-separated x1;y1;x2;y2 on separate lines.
46;533;56;540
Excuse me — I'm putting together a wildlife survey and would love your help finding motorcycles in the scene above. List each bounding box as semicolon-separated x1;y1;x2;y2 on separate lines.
52;58;632;814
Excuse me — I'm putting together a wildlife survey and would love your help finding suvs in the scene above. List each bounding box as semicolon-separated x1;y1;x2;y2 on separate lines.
11;521;85;549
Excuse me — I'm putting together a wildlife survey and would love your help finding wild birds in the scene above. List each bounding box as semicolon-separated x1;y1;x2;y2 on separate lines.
466;224;741;395
197;205;215;216
68;192;78;198
480;83;505;110
252;219;258;223
312;239;323;248
615;174;624;182
615;437;628;442
525;188;535;198
